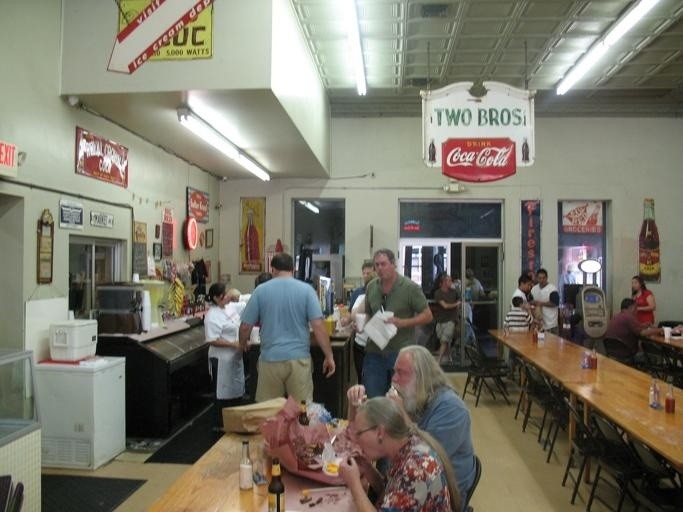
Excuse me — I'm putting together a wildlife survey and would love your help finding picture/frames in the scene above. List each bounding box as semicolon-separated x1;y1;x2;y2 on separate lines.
205;228;213;248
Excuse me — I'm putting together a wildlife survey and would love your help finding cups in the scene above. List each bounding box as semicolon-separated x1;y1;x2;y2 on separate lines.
663;327;672;341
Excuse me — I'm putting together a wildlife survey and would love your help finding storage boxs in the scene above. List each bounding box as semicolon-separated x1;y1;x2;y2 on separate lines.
47;318;97;362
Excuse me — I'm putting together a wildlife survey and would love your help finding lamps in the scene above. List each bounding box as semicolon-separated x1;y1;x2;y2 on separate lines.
175;107;271;184
338;0;372;97
554;0;663;99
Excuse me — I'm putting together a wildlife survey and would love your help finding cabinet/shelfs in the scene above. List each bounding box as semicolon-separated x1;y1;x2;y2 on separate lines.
0;347;43;511
97;308;211;438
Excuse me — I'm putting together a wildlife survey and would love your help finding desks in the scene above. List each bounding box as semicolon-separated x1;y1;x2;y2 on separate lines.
246;323;355;419
145;417;378;511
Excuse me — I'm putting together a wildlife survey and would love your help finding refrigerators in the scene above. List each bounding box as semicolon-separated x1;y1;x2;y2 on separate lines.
33;355;126;470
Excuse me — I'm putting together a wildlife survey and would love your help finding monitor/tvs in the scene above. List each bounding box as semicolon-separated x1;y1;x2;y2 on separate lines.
563;284;598;309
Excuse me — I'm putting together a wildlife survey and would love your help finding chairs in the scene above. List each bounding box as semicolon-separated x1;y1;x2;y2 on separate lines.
462;321;682;463
561;396;682;507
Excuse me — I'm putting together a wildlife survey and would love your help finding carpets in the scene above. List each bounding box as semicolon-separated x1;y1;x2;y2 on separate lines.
41;472;149;510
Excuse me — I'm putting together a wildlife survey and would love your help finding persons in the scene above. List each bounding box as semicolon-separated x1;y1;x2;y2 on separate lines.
336;396;463;511
202;282;246;432
526;268;560;338
604;298;664;366
360;248;434;400
673;323;682;336
510;274;544;333
433;271;462;369
224;259;379;382
629;275;656;328
238;253;337;405
345;343;476;511
502;296;534;381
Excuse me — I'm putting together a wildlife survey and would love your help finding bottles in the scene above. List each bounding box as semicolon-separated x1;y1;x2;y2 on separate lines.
298;399;309;425
664;380;675;413
648;375;660;408
237;438;253;491
274;239;283;255
580;346;597;369
265;457;285;512
243;210;260;264
585;205;601;225
532;329;537;342
637;197;661;285
